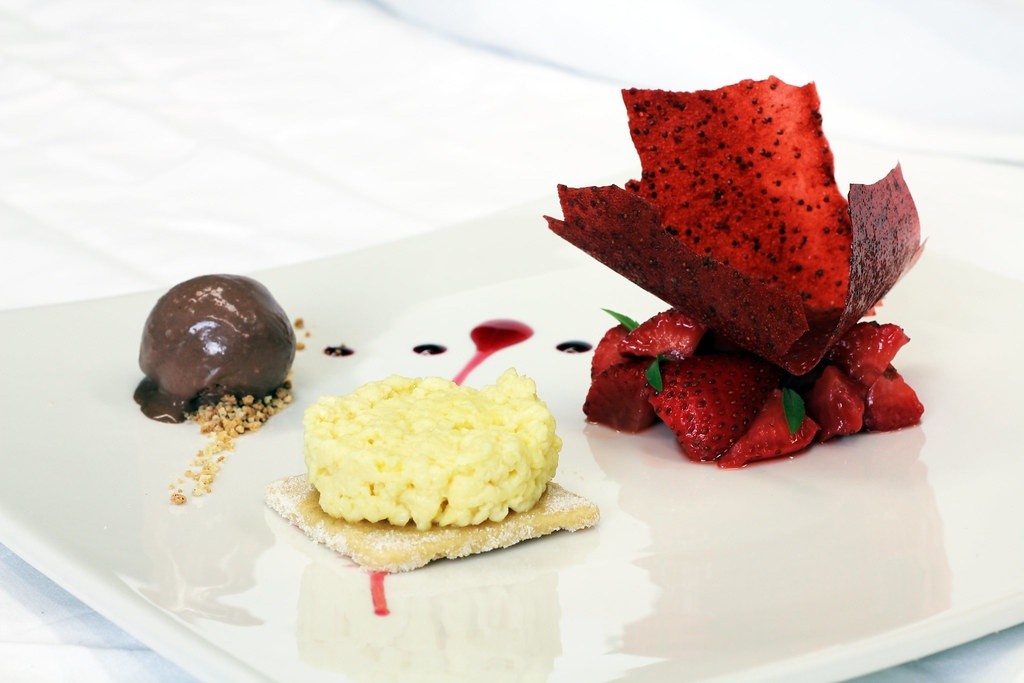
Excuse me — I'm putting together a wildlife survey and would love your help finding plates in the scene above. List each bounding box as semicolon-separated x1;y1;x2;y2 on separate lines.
0;199;1022;683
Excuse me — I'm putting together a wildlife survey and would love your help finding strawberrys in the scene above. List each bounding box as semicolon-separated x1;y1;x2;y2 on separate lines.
577;305;926;466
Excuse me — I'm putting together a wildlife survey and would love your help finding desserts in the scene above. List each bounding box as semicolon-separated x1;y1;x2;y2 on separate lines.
262;365;598;572
